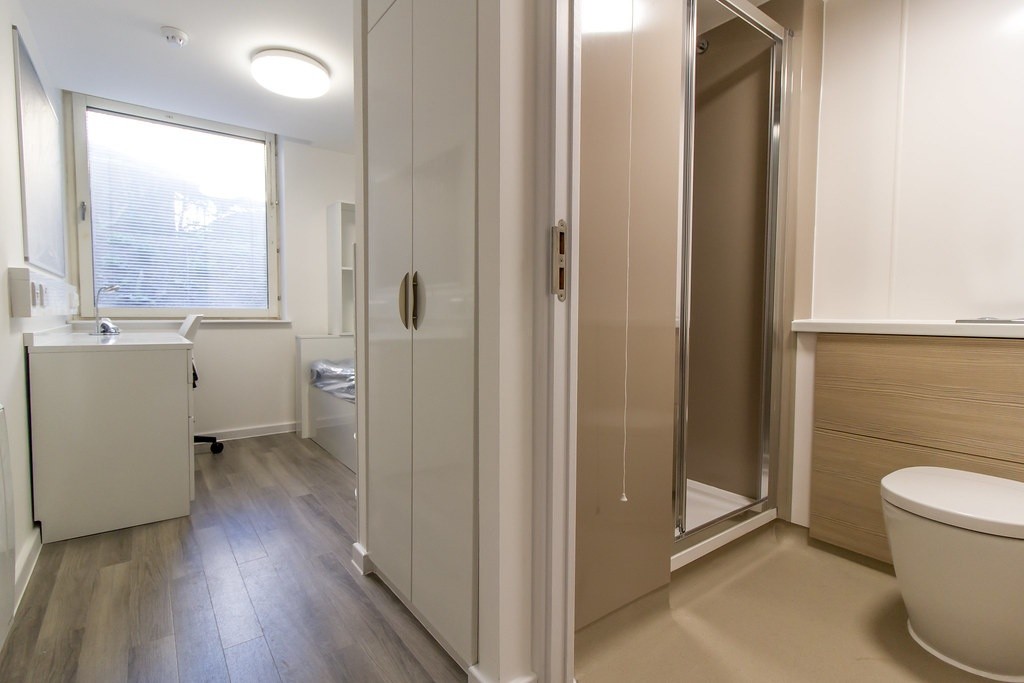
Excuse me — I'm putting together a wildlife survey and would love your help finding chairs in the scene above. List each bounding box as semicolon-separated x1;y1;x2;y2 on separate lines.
179;313;225;455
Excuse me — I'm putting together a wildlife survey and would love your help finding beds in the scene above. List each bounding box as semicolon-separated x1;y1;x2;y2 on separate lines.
295;334;357;477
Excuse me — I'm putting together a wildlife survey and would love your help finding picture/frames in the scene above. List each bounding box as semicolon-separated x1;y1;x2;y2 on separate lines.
12;25;69;278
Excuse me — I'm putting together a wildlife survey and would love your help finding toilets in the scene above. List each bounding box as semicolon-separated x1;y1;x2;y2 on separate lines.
880;467;1024;682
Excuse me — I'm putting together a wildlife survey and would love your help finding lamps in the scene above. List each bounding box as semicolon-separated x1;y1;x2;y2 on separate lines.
89;285;121;336
250;48;331;100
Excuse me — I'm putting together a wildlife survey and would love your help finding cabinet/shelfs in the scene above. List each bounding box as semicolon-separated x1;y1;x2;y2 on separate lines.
365;0;478;674
327;201;357;336
25;322;196;545
